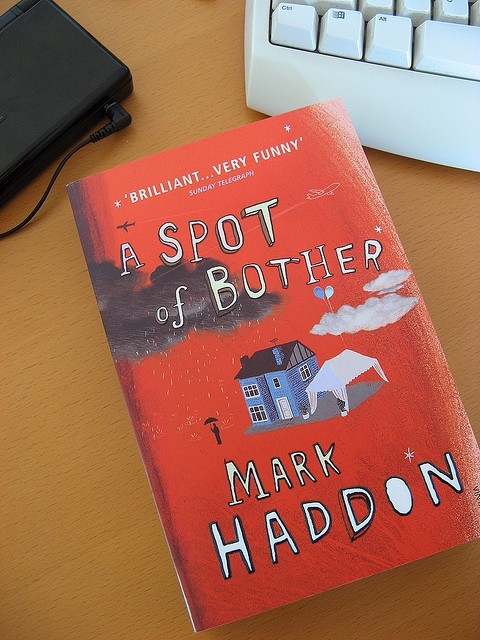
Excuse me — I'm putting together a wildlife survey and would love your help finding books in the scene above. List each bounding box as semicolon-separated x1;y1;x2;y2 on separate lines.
64;94;479;633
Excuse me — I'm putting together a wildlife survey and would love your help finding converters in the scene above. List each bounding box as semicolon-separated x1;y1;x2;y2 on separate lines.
0;0;133;208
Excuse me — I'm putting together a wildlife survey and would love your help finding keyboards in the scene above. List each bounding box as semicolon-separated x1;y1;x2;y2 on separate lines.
245;1;480;172
